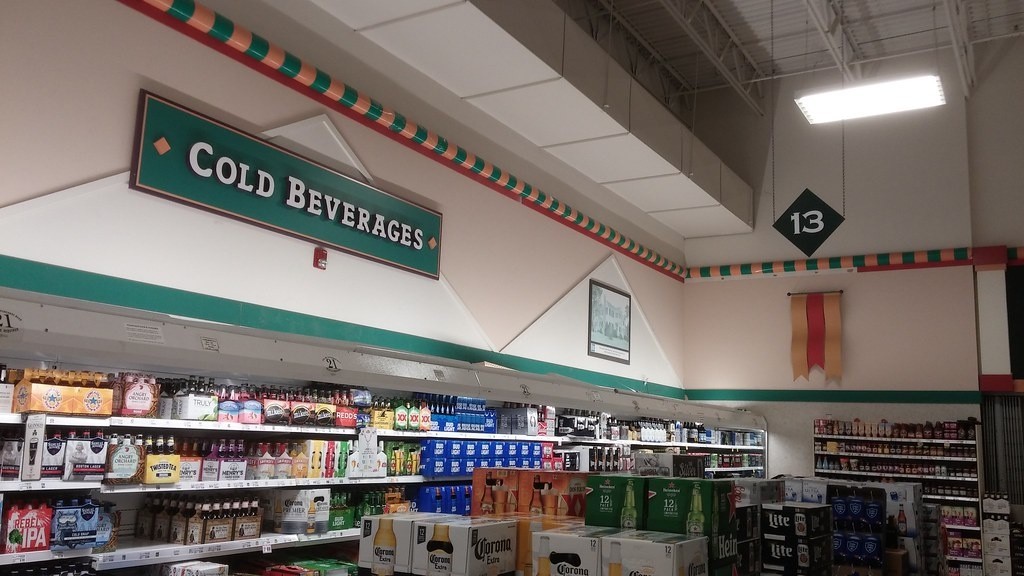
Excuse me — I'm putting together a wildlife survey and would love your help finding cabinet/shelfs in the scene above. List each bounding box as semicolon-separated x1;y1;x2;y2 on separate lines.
0;299;768;576
811;420;986;576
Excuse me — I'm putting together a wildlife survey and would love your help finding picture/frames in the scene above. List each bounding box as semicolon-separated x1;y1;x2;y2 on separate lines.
588;278;630;365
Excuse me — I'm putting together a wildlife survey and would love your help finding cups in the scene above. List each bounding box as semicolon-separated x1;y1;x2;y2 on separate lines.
540;489;558;517
492;486;507;516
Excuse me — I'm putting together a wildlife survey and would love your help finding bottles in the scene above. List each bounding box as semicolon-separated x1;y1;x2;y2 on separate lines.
138;496;261;546
711;480;720;533
529;474;542;513
710;534;720;576
451;487;457;513
900;539;905;550
686;482;705;536
0;497;120;554
534;535;552;576
435;488;441;513
608;541;622;576
306;501;316;534
898;504;908;535
677;544;685;576
621;479;637;528
464;486;471;516
330;486;406;530
273;500;282;533
947;564;982;576
501;400;707;443
983;488;1024;576
426;523;453;576
371;519;396;576
481;472;493;516
0;361;484;479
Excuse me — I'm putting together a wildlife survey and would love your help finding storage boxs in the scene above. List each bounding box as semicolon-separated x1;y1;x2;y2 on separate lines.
1;369;943;575
941;496;1024;576
